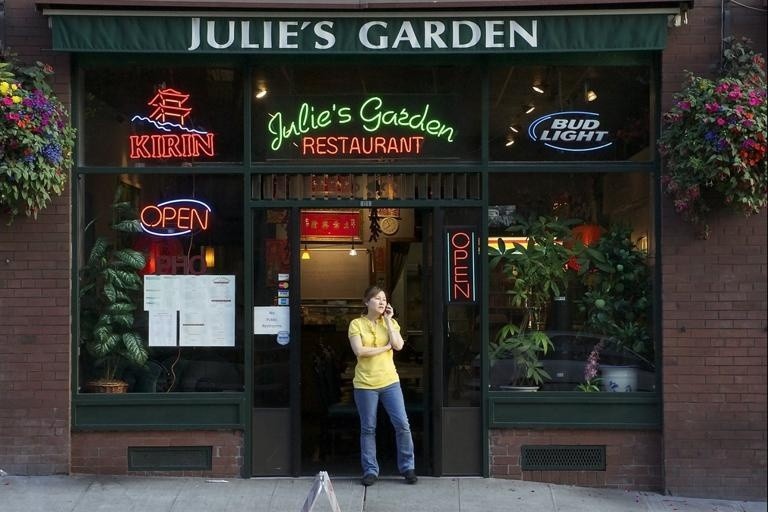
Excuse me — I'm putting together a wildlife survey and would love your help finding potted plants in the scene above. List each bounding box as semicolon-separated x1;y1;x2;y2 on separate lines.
576;219;650;393
487;211;568;393
79;184;146;393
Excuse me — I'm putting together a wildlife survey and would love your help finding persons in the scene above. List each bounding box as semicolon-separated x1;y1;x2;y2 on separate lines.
349;286;418;487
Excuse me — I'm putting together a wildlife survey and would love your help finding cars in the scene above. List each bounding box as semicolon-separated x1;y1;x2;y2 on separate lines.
452;330;654;401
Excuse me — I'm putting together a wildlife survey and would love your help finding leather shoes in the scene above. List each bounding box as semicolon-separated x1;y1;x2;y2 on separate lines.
362;474;377;486
404;470;417;484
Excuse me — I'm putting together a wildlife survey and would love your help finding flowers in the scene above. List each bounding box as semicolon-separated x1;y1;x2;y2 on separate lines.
655;32;768;238
0;42;78;227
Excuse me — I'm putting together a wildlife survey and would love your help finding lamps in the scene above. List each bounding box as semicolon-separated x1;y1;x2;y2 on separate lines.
505;73;548;150
204;244;217;268
254;76;267;98
349;209;358;256
300;206;312;259
581;83;598;103
